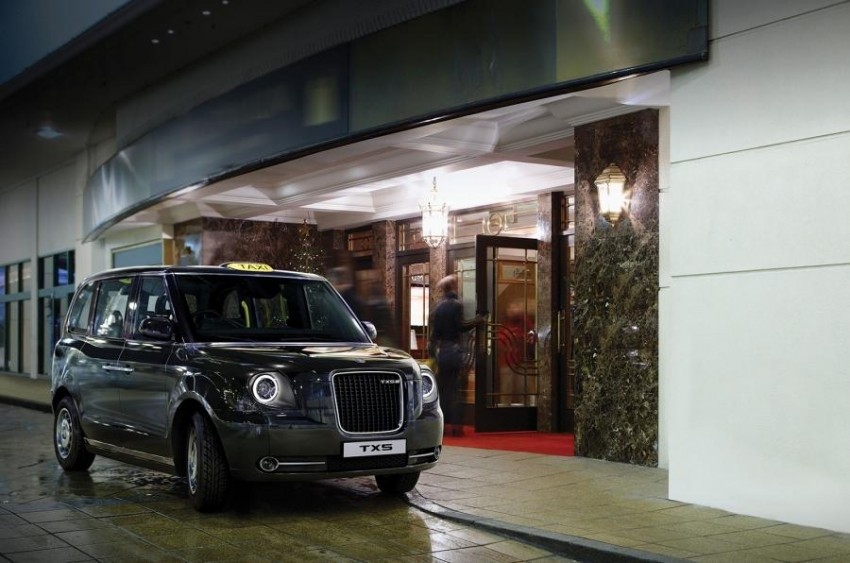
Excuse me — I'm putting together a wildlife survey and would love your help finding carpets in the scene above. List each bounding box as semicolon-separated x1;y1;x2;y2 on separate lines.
443;425;575;456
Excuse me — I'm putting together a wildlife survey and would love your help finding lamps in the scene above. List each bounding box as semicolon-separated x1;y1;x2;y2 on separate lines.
419;175;450;248
595;163;627;228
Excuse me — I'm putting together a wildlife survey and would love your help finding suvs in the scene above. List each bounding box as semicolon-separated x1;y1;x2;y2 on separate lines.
51;261;445;513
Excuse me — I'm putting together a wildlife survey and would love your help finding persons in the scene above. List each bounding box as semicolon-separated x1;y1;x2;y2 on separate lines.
429;274;487;435
340;286;363;324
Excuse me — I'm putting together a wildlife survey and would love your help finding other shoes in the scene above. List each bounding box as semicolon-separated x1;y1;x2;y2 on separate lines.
452;429;464;435
